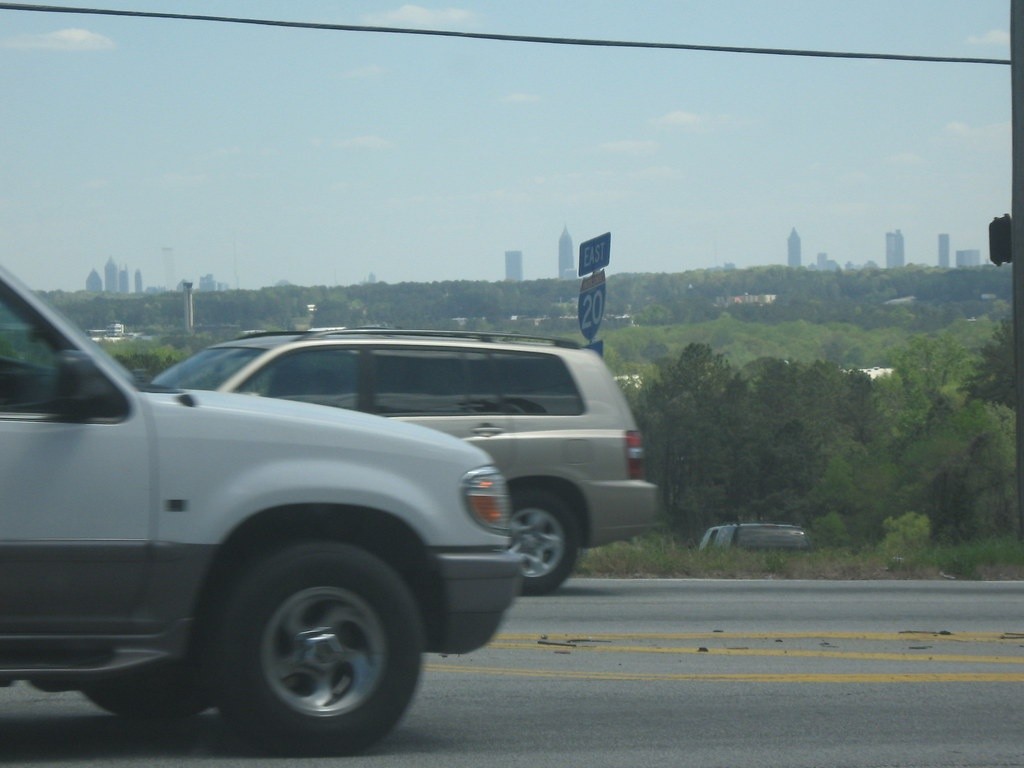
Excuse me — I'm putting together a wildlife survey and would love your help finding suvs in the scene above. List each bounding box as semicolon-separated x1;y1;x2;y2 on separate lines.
1;260;524;755
151;329;666;597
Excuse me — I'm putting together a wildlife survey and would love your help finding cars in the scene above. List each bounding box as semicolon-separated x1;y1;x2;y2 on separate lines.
698;522;812;559
